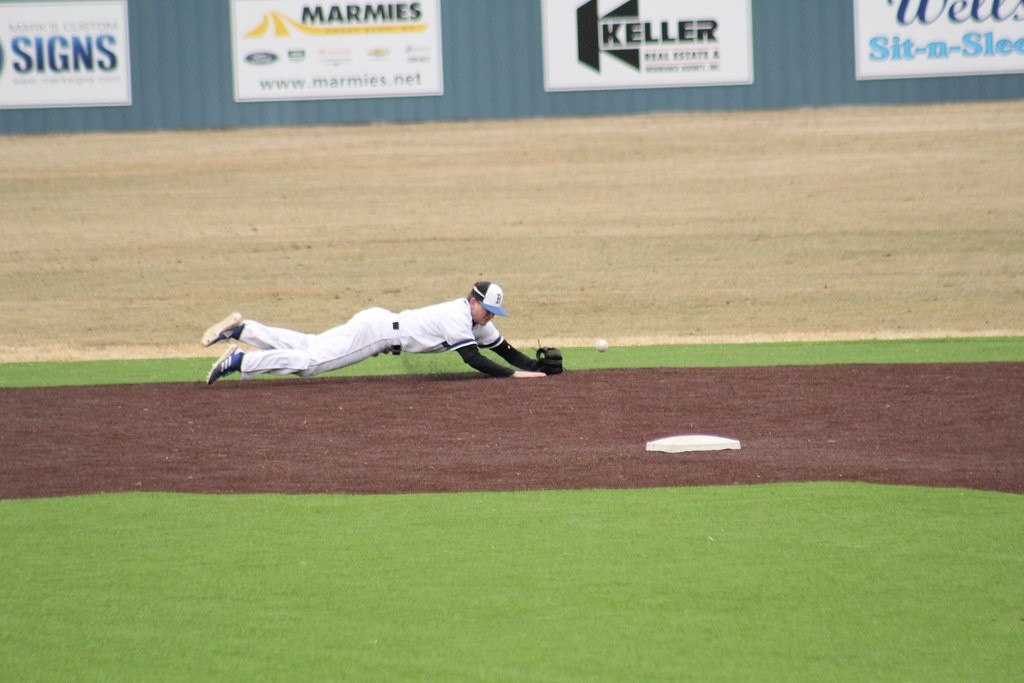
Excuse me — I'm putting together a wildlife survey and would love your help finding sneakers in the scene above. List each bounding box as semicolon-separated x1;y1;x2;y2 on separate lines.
206;343;245;385
202;313;244;347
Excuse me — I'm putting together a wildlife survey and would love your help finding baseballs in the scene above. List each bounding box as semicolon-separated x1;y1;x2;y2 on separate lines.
595;338;608;352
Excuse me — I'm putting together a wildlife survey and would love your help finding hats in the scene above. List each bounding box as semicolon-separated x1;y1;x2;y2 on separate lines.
470;282;509;317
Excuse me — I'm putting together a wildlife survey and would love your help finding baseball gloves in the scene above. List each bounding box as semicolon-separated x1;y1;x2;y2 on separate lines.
531;339;562;374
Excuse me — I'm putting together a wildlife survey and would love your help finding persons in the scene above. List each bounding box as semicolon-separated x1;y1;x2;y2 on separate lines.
203;281;563;385
